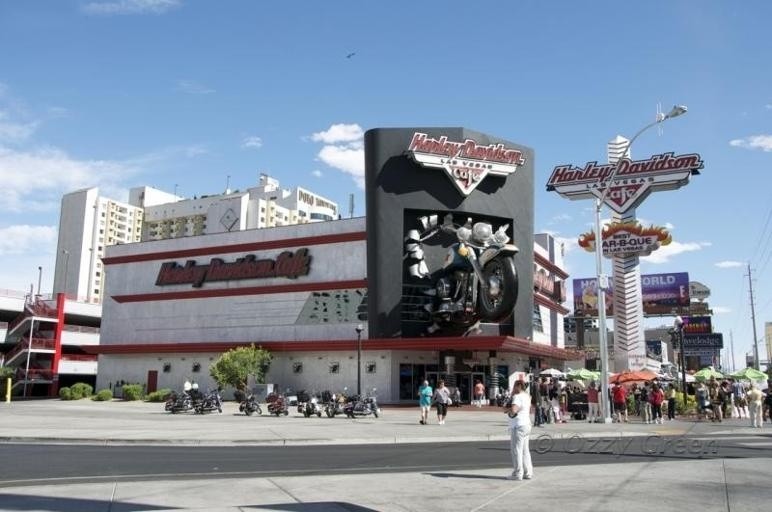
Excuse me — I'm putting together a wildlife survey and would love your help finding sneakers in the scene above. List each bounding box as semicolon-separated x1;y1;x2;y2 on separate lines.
505;473;532;480
438;420;445;425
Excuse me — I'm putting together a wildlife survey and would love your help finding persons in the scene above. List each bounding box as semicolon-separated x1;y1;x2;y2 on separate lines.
183;379;198;393
508;380;533;481
452;386;461;408
475;379;484;407
417;380;450;424
533;376;772;428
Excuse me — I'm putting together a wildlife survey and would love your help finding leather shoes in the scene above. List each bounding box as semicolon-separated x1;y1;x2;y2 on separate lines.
420;419;428;425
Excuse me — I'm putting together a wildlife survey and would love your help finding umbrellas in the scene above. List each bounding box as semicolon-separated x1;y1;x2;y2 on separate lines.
540;367;768;385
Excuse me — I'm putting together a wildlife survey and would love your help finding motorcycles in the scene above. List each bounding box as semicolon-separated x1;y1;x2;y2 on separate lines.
418;216;520;340
163;379;382;420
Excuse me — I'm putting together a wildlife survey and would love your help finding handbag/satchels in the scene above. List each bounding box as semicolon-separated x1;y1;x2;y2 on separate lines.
504;400;517;418
448;397;453;405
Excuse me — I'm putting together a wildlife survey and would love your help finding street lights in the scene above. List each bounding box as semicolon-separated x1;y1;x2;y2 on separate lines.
591;99;690;421
36;265;41;294
674;314;688;408
61;248;69;293
354;322;364;396
